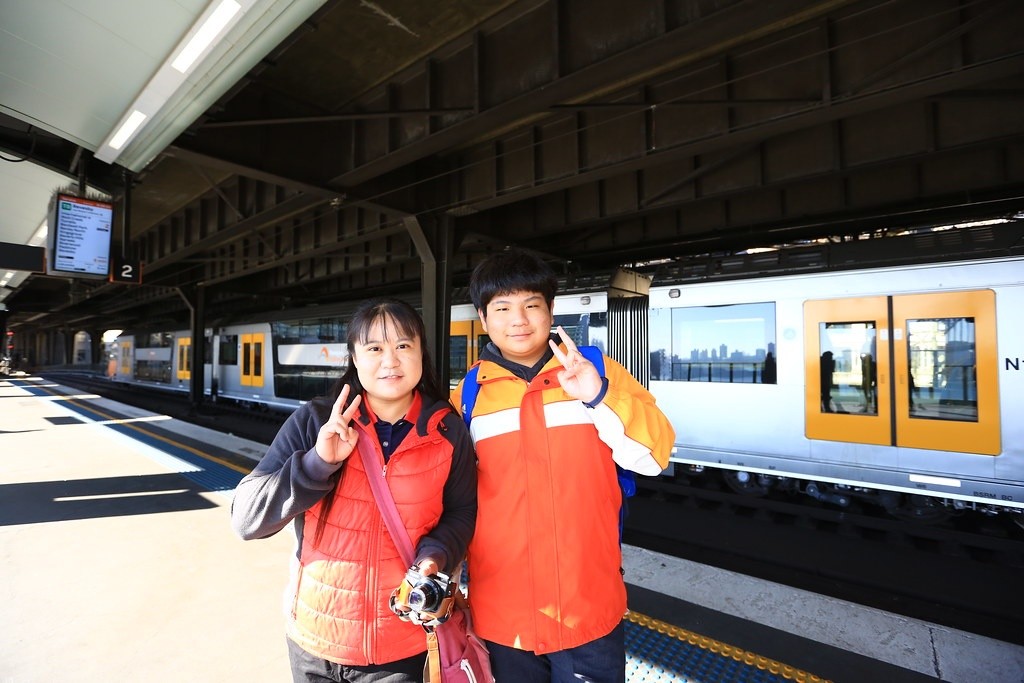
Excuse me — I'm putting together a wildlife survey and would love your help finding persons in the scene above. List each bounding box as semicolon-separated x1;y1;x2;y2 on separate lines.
820;351;835;412
445;250;677;683
861;354;915;411
760;351;776;384
229;297;478;683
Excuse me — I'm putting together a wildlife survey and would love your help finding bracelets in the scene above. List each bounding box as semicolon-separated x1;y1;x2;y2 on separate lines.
581;377;609;409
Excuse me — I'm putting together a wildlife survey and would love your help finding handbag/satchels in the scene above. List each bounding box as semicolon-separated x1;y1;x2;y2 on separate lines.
423;606;493;683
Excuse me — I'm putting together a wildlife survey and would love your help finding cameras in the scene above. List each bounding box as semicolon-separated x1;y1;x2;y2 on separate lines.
402;565;457;621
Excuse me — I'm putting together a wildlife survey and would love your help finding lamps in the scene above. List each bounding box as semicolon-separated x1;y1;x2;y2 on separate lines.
94;0;262;166
0;218;51;290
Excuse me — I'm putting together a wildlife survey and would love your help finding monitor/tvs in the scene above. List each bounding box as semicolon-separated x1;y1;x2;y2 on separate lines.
51;192;115;277
